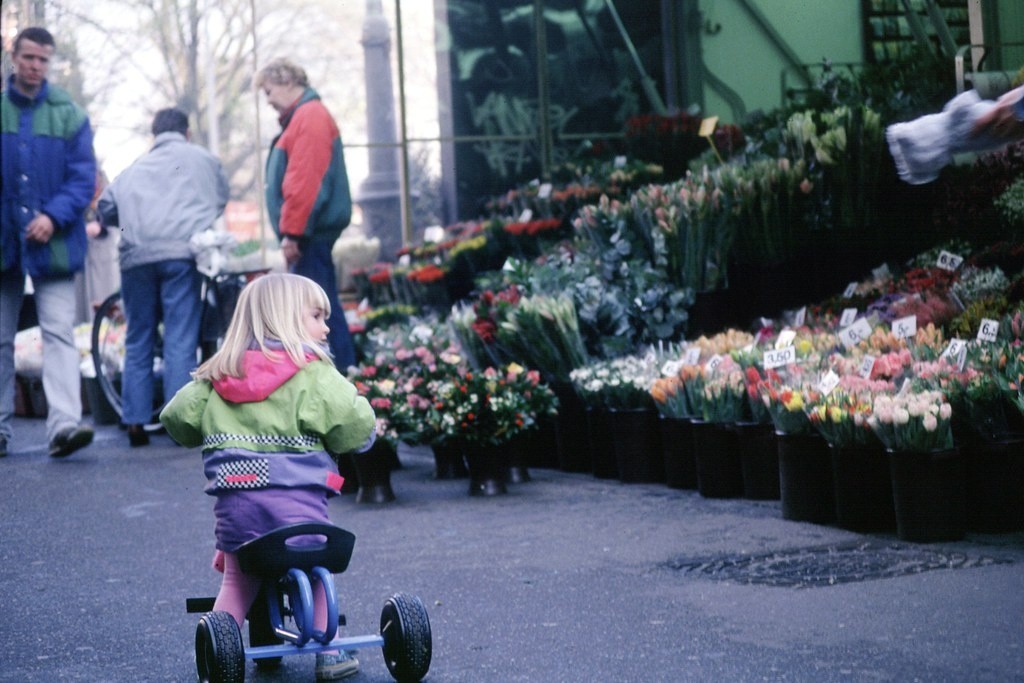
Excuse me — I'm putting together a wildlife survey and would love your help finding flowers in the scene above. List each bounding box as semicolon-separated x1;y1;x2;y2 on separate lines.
331;42;1023;447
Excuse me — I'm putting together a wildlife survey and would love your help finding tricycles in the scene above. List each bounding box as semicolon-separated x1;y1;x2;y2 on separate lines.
186;511;435;683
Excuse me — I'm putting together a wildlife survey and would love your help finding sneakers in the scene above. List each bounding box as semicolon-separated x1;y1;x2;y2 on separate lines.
313;651;362;679
47;427;94;457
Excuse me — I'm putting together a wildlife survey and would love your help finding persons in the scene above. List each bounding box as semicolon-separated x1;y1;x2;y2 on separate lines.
0;26;97;459
159;270;376;679
253;54;359;371
96;108;232;445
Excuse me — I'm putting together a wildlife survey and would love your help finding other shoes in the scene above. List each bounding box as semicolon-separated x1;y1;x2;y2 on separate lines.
124;424;148;444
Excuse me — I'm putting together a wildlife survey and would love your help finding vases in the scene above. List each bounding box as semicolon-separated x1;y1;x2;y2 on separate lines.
435;402;1024;543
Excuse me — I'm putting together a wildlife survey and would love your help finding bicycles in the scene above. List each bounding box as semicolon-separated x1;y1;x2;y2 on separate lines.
87;262;282;438
